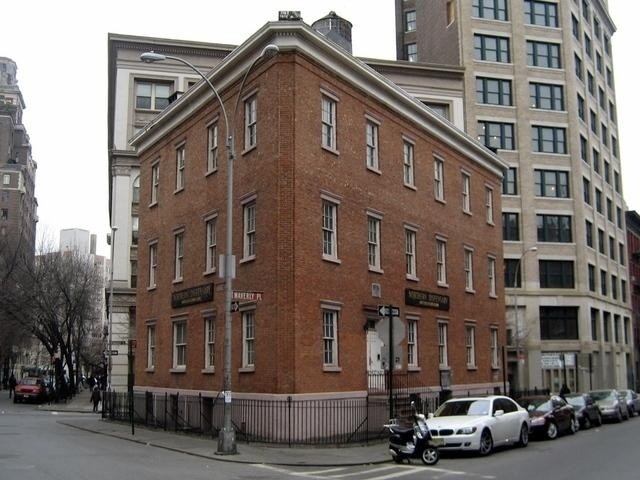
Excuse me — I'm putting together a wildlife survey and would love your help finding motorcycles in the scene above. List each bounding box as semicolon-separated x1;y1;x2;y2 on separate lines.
383;399;441;467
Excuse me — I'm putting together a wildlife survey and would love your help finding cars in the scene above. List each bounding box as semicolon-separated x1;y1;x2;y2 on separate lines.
45;380;54;394
424;388;639;457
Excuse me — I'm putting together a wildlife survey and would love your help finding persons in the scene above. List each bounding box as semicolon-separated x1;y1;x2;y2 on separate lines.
90;388;102;413
9;373;17;398
2;375;8;391
87;374;95;394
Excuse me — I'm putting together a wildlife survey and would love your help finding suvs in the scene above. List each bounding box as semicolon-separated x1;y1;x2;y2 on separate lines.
13;375;46;405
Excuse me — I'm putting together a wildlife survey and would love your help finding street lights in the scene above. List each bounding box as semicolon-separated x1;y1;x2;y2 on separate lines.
137;43;281;455
513;247;539;395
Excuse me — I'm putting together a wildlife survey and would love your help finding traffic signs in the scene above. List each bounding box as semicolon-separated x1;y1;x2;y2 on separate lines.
232;290;265;302
378;305;400;318
229;300;240;312
102;349;118;356
